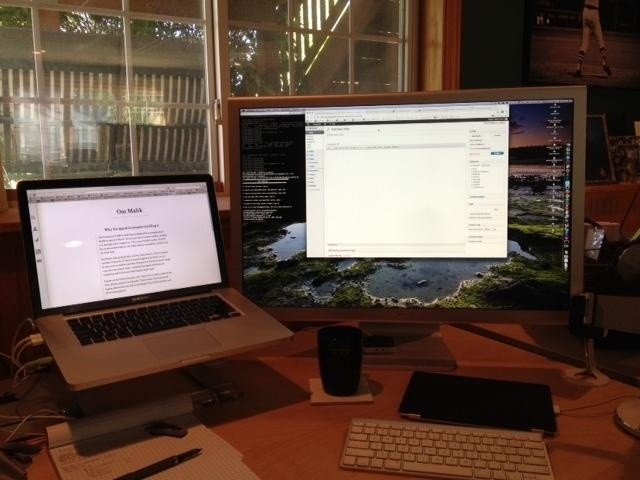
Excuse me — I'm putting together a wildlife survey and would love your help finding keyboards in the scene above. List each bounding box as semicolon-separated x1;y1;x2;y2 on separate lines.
339;417;554;479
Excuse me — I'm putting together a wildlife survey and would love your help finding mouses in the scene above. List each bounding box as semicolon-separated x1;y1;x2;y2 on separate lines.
614;398;639;437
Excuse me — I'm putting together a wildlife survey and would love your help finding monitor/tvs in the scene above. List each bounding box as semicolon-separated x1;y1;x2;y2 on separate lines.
225;85;587;371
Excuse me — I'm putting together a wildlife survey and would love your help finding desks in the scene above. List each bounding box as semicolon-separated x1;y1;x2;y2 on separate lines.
585;179;639;242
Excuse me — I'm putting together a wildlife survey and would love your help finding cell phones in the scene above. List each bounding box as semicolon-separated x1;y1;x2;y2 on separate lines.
363;335;396;353
399;371;558;434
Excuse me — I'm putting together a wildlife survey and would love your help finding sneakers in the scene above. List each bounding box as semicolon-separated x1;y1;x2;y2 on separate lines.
575;62;583;77
600;59;612;76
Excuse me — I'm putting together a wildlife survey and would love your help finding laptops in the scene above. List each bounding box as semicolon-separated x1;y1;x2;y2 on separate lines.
17;172;296;392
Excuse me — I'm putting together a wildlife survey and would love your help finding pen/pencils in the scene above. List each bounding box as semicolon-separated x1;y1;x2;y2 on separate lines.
111;449;204;480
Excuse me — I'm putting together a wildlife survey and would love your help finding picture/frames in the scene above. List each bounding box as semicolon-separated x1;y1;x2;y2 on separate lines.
585;114;618;185
521;0;640;98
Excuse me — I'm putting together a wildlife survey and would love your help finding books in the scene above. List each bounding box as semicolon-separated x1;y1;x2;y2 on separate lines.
46;392;259;480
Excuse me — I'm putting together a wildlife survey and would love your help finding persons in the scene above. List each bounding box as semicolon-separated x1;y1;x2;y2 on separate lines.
574;0;614;77
